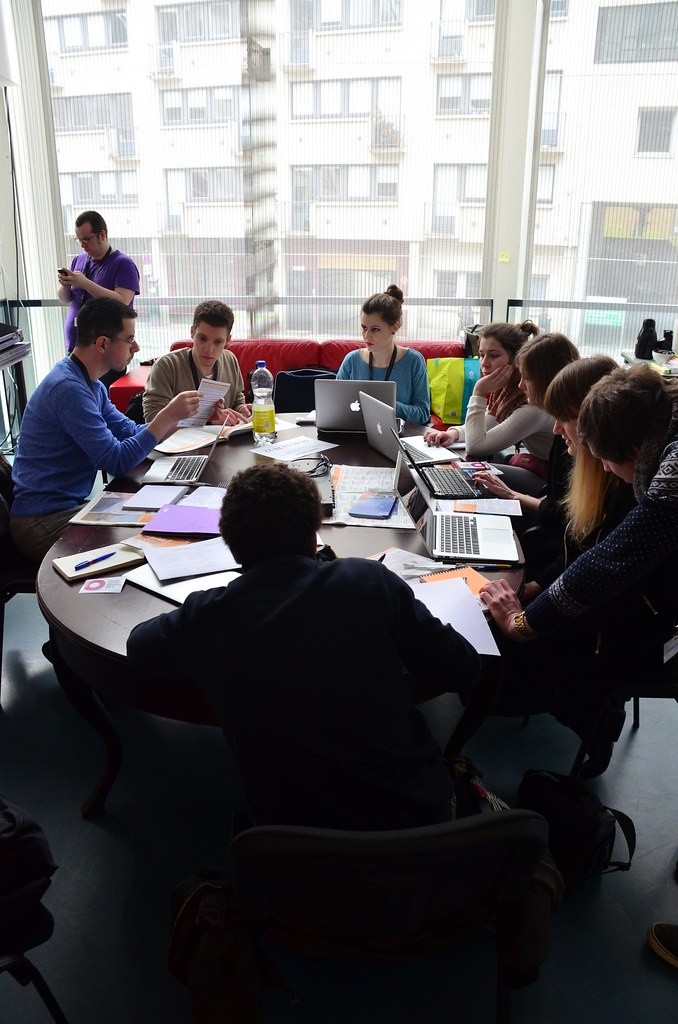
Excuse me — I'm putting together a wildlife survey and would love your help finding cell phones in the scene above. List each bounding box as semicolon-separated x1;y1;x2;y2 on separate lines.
57;269;69;276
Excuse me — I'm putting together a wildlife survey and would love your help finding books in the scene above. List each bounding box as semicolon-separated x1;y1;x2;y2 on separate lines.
452;461;490;469
420;566;491;612
52;543;147;581
154;424;253;454
123;485;190;511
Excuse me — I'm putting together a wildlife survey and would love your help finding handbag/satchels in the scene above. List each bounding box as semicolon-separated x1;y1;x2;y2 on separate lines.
426;354;482;426
168;870;262;1024
273;366;338;413
518;765;637;880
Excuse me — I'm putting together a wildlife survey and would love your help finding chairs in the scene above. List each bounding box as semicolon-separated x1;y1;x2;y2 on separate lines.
216;807;544;1024
0;451;52;697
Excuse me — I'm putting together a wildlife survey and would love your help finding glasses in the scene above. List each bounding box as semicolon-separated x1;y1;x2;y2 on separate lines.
93;335;136;348
74;230;104;244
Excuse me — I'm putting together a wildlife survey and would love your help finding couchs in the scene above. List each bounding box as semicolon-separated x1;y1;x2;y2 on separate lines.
108;337;471;430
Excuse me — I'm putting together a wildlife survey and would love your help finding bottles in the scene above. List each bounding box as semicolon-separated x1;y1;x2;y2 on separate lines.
250;360;276;444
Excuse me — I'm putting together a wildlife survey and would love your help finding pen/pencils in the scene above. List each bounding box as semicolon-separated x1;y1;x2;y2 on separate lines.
177;482;211;486
378;554;386;562
75;552;116;570
456;563;512;569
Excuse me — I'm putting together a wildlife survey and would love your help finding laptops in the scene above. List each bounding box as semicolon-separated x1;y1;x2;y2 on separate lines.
142;414;230;483
315;379;518;565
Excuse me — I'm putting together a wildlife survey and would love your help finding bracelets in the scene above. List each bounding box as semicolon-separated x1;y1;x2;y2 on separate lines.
514;612;534;642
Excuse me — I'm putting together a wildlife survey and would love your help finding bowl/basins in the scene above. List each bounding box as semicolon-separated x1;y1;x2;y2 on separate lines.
652;350;676;366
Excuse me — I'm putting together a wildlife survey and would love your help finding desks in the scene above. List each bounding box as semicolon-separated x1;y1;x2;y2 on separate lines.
621;351;678;378
37;414;525;823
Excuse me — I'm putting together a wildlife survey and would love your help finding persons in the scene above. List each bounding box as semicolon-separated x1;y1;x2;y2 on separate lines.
142;300;253;426
56;210;140;389
336;284;431;424
12;295;225;679
129;465;482;831
423;322;626;778
479;367;678;967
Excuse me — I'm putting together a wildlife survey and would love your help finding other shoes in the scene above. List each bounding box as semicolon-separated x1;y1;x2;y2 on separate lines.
647;919;678;970
